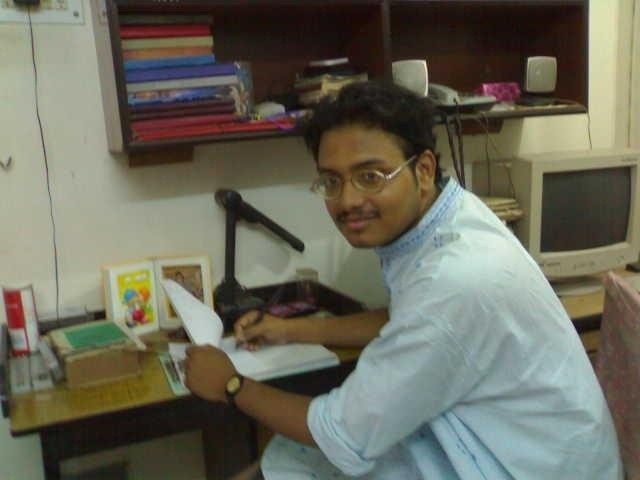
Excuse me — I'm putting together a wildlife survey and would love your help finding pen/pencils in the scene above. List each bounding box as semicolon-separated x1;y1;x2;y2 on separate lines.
235;289;280;349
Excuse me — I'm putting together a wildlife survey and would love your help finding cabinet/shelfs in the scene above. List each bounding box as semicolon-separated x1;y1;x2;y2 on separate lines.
90;0;590;169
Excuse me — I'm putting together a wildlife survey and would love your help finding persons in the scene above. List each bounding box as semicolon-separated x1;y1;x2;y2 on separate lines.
185;80;626;479
176;271;192;293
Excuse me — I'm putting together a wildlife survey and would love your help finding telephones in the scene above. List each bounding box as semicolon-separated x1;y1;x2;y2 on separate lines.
428;83;497;119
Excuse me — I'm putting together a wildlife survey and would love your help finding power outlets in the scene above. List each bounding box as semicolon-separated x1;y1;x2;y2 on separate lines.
0;0;84;25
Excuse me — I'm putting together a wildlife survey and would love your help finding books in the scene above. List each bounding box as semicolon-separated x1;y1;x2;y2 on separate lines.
162;278;339;395
48;320;146;390
112;13;288;142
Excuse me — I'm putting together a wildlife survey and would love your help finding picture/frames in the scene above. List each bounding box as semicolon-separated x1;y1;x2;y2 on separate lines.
154;256;213;330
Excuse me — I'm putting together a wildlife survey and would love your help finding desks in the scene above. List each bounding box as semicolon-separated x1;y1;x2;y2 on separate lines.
9;287;603;480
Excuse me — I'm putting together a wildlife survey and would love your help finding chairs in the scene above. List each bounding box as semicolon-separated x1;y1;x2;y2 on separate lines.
596;269;640;480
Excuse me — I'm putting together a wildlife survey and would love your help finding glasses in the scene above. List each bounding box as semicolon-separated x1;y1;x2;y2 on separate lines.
310;155;418;200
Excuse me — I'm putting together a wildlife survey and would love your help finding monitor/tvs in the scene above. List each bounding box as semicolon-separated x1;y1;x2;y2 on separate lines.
470;148;639;295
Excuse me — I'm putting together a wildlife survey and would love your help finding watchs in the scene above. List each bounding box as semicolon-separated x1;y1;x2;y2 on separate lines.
226;374;244;409
